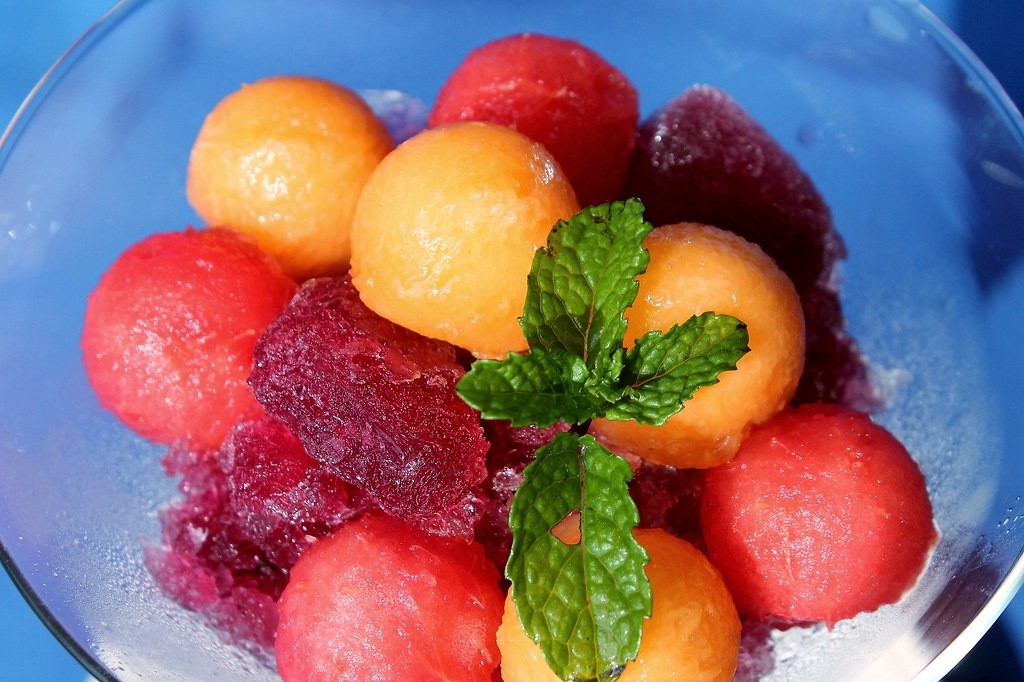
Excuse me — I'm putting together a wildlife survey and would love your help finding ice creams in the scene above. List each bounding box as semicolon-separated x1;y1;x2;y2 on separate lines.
80;34;939;682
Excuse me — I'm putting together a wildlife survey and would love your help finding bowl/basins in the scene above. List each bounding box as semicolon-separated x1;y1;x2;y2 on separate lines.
1;0;1024;681
